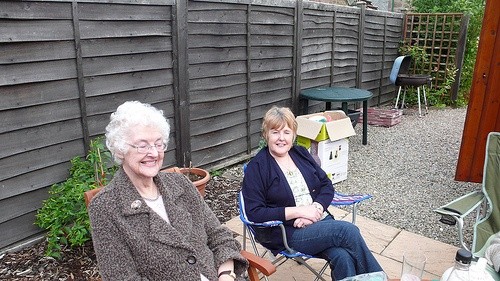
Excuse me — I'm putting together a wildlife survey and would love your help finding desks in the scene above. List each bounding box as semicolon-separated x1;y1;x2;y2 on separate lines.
299;88;375;146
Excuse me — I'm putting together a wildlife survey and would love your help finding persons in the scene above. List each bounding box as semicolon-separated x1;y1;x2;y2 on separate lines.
88;100;250;281
242;106;390;281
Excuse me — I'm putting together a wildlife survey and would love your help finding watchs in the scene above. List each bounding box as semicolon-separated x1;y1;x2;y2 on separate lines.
218;270;237;279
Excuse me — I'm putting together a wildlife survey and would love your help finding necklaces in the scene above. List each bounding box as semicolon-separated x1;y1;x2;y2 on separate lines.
272;153;293;176
123;164;160;201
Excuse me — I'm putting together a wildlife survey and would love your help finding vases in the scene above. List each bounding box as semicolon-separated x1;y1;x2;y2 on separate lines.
180;168;210;198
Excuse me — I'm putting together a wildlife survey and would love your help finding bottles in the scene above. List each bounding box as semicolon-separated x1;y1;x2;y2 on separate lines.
439;248;479;281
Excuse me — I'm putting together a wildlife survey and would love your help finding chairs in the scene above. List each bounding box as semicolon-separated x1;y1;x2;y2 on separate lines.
238;162;372;281
83;187;277;281
434;132;500;258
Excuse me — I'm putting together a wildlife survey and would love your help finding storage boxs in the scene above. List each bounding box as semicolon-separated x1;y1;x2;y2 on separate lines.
296;112;357;186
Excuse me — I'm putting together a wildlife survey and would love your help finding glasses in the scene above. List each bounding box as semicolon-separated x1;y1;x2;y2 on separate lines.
125;143;169;154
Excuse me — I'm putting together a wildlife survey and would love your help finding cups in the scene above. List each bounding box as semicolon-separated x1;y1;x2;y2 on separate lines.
401;250;427;281
